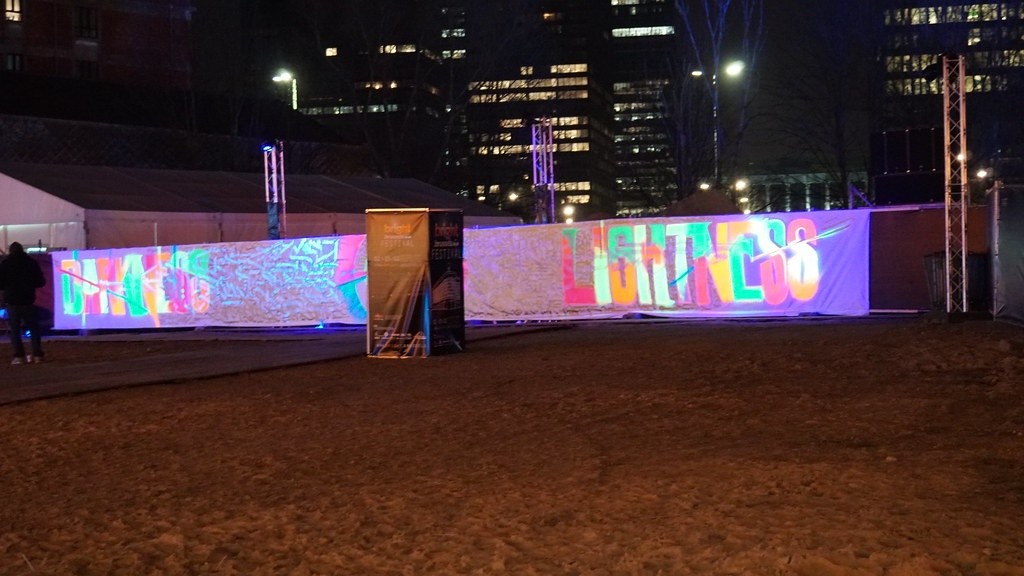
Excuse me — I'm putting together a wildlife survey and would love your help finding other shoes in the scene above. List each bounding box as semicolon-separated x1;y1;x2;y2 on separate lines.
11;357;23;365
34;355;44;364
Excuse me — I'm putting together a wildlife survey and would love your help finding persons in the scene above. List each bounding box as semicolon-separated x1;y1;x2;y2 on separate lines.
1;241;48;364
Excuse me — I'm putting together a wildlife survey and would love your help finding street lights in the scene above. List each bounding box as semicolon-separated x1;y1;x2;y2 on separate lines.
273;72;297;110
690;59;745;190
